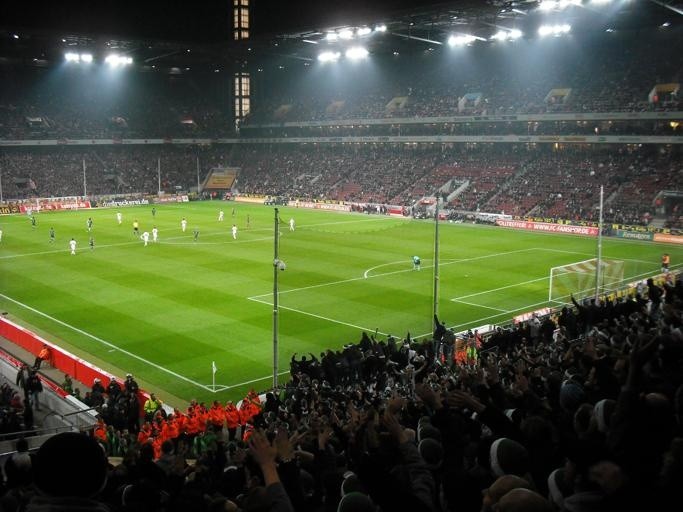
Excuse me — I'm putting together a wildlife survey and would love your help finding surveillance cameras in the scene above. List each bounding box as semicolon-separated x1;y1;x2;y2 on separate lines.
280;263;286;272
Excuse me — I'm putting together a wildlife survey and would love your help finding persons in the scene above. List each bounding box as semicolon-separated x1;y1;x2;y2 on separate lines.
69;238;77;256
0;314;683;511
132;218;139;236
152;207;156;216
289;217;295;231
142;226;158;247
511;40;683;228
218;208;250;240
193;224;200;241
235;42;524;223
50;226;56;245
1;41;233;207
88;237;96;249
489;253;683;368
181;217;187;232
117;211;123;225
411;255;422;272
31;216;38;231
88;217;93;229
